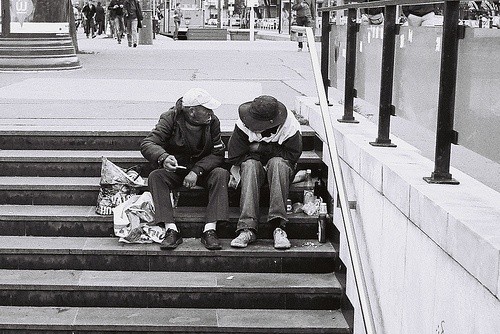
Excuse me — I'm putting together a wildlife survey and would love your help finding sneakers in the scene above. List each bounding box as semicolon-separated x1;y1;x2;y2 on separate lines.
273;228;290;248
201;229;222;249
230;228;255;247
160;227;182;248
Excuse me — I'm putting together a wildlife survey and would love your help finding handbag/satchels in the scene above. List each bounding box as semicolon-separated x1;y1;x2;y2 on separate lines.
95;155;145;215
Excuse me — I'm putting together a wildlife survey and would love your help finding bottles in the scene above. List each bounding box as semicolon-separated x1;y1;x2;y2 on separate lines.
314;169;326;202
303;169;315;205
320;202;328;215
286;199;293;214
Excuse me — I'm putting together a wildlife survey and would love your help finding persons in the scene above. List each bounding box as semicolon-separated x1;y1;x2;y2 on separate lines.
173;3;183;39
227;95;302;248
364;0;434;26
292;0;315;52
81;0;104;38
140;89;230;249
108;0;125;44
122;0;143;47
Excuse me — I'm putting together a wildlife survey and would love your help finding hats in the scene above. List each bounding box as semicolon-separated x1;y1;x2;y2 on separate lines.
182;88;221;110
238;95;287;132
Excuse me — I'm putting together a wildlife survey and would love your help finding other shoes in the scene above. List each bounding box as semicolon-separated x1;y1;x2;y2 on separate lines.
134;43;137;47
308;48;310;51
92;35;96;38
129;44;132;46
298;48;302;51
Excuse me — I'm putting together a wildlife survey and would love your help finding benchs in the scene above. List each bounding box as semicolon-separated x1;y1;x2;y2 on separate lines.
186;28;227;41
229;30;259;41
